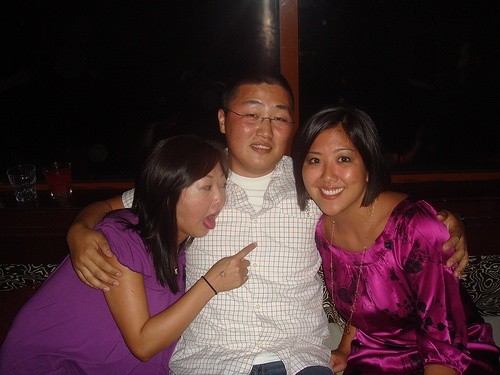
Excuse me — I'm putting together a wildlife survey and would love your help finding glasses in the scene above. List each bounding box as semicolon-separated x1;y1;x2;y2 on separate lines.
225;108;295;129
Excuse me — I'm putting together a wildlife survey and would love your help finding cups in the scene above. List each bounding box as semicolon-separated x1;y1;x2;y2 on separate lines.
43;160;72;201
7;164;38;206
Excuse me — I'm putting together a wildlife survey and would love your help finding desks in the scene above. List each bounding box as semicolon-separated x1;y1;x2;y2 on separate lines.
0;182;500;252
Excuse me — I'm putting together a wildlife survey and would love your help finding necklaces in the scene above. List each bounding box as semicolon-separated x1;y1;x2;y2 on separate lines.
331;195;377;335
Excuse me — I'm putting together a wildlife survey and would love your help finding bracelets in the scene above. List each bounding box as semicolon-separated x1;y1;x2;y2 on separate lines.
200;276;218;295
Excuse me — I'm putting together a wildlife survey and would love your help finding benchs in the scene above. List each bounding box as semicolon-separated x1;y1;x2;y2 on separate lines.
0;253;500;347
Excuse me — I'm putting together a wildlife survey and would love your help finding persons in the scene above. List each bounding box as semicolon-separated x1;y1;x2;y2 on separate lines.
0;134;258;375
66;67;469;375
292;106;500;375
